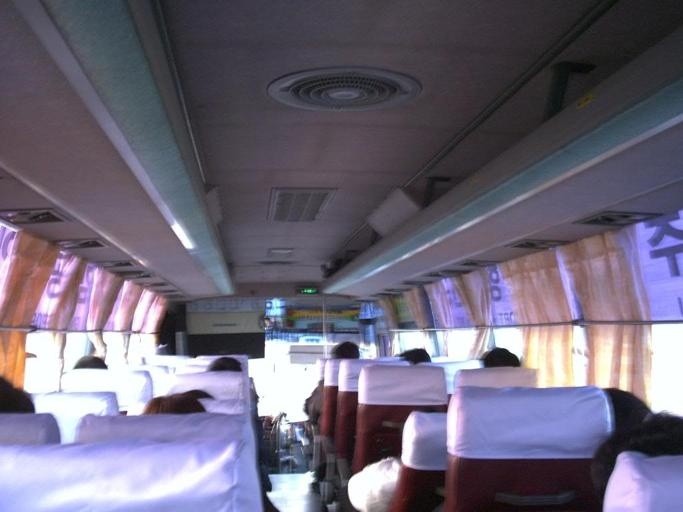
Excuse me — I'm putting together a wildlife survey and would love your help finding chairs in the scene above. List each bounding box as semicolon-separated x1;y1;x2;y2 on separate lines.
0;351;268;511
307;356;683;512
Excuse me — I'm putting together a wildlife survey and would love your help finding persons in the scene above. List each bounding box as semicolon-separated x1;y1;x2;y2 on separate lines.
592;412;682;504
74;355;104;369
398;347;432;363
140;396;203;412
204;357;243;374
333;442;403;510
301;342;358;424
0;375;34;412
601;386;653;443
183;387;220;400
482;347;522;364
